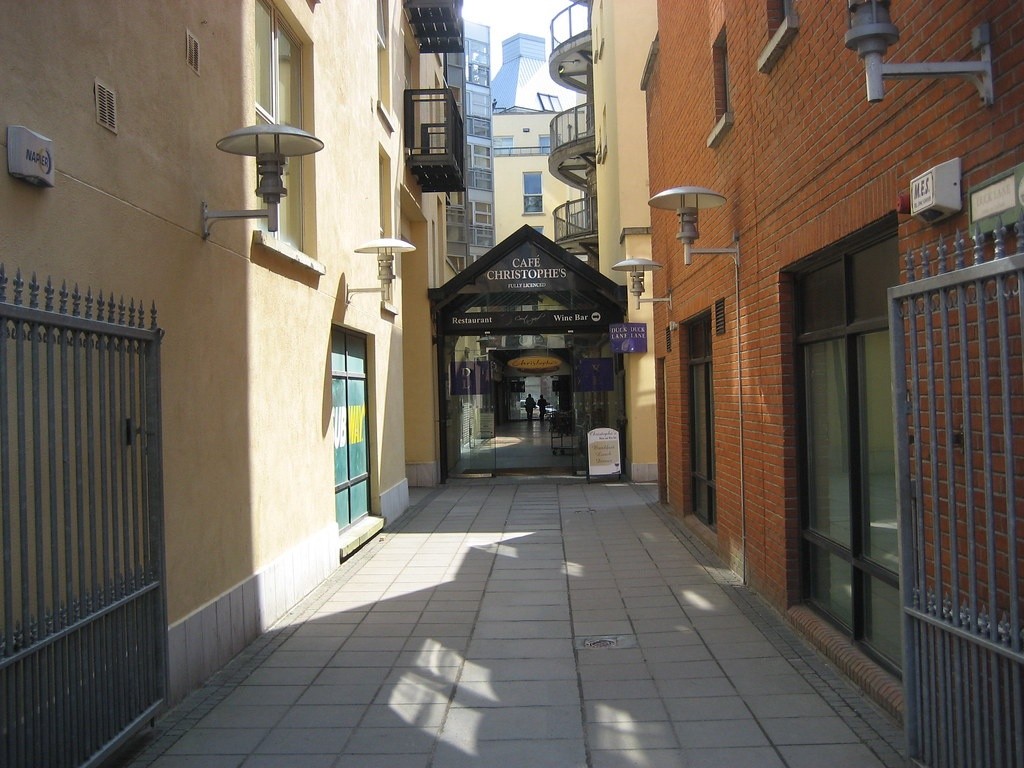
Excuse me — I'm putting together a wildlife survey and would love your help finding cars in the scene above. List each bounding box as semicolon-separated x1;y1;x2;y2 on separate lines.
520;400;557;418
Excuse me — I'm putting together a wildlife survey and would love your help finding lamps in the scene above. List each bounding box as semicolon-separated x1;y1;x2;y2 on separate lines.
203;124;324;239
648;186;741;271
843;0;993;107
558;65;565;73
523;128;529;132
611;257;673;310
344;237;417;306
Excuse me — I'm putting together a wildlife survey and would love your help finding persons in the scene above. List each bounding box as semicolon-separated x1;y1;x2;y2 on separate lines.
537;395;547;421
526;394;534;422
